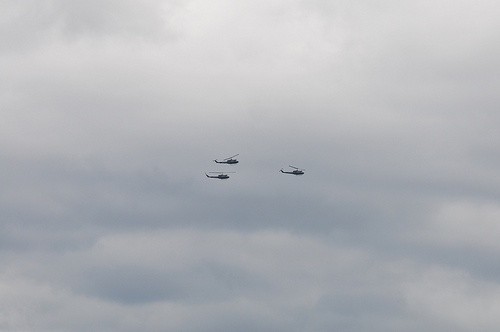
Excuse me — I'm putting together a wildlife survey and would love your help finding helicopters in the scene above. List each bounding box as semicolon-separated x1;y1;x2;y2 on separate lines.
205;171;234;179
214;154;240;164
280;165;304;176
